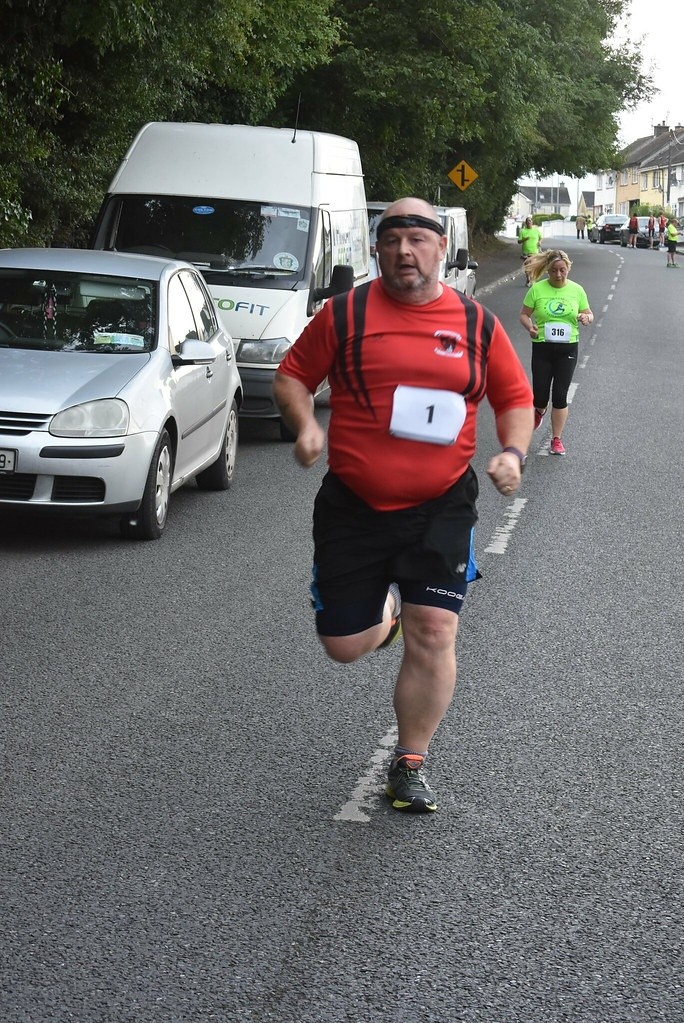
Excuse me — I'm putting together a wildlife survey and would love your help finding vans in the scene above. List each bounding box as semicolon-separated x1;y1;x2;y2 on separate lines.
363;201;481;302
91;89;373;440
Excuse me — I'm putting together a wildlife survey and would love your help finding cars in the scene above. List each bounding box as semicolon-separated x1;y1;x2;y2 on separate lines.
0;247;241;542
664;216;684;245
586;214;630;244
618;216;667;250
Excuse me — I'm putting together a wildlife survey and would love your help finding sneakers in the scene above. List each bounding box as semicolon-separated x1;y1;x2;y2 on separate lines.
384;749;438;810
534;408;547;430
549;436;566;456
373;582;400;649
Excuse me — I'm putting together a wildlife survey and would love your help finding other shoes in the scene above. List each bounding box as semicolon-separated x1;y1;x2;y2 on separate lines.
627;244;665;249
526;280;531;287
673;263;680;268
667;263;673;268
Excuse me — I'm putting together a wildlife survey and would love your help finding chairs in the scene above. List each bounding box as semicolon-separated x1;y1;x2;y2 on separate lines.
0;279;45;340
80;299;136;340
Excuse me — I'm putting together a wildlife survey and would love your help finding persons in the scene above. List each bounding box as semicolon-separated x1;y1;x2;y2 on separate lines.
667;219;682;269
628;213;640;249
519;249;595;456
585;215;592;239
517;218;542;286
657;212;666;248
270;196;533;814
648;211;655;249
575;213;585;239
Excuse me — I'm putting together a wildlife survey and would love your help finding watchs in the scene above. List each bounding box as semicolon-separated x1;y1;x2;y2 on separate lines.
501;447;527;474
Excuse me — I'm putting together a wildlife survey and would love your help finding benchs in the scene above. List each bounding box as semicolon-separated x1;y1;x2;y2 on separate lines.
39;283;151;348
